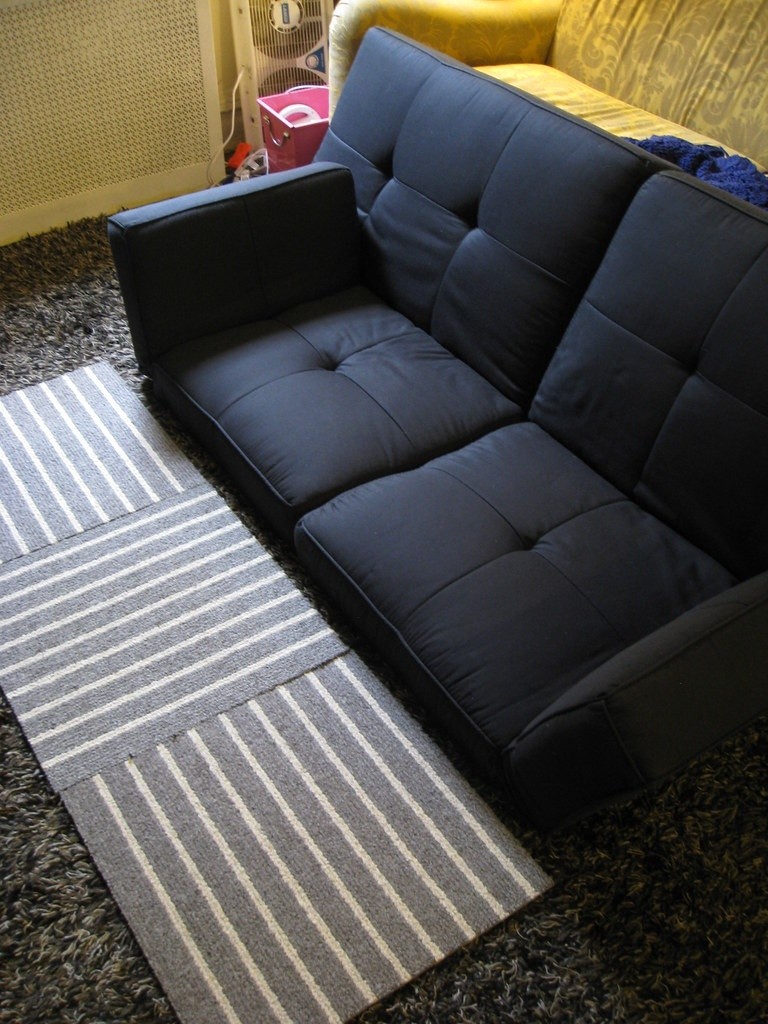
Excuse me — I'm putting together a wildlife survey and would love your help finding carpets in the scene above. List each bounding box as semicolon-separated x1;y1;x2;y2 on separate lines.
0;360;557;1024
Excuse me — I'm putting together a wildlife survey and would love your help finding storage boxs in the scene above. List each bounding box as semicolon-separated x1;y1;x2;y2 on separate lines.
256;85;331;173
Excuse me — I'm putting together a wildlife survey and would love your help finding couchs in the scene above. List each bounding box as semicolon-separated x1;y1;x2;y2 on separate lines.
104;29;768;831
327;0;768;210
